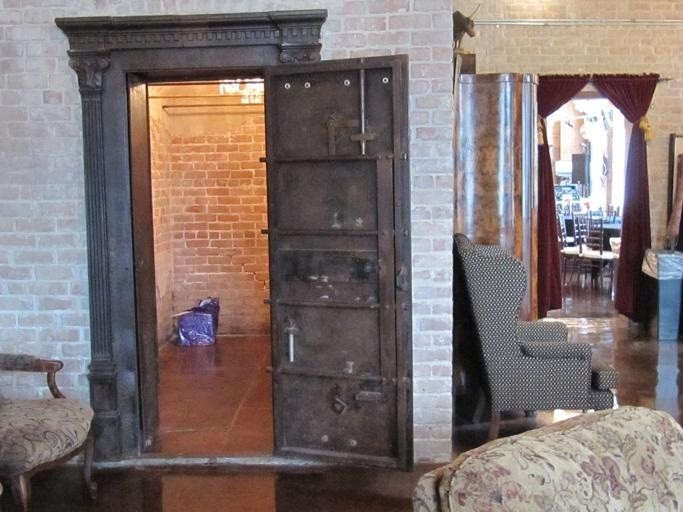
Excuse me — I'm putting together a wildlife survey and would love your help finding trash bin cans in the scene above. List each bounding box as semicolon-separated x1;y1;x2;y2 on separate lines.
645;249;683;341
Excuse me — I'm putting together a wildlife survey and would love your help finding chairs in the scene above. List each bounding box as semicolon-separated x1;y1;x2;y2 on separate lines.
452;232;622;443
556;206;624;298
0;352;100;512
410;401;683;511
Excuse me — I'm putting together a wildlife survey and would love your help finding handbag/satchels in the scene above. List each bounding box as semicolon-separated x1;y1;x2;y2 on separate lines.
172;311;215;347
190;296;220;332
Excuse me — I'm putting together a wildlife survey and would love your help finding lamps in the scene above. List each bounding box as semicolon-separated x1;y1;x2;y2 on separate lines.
214;77;267;105
